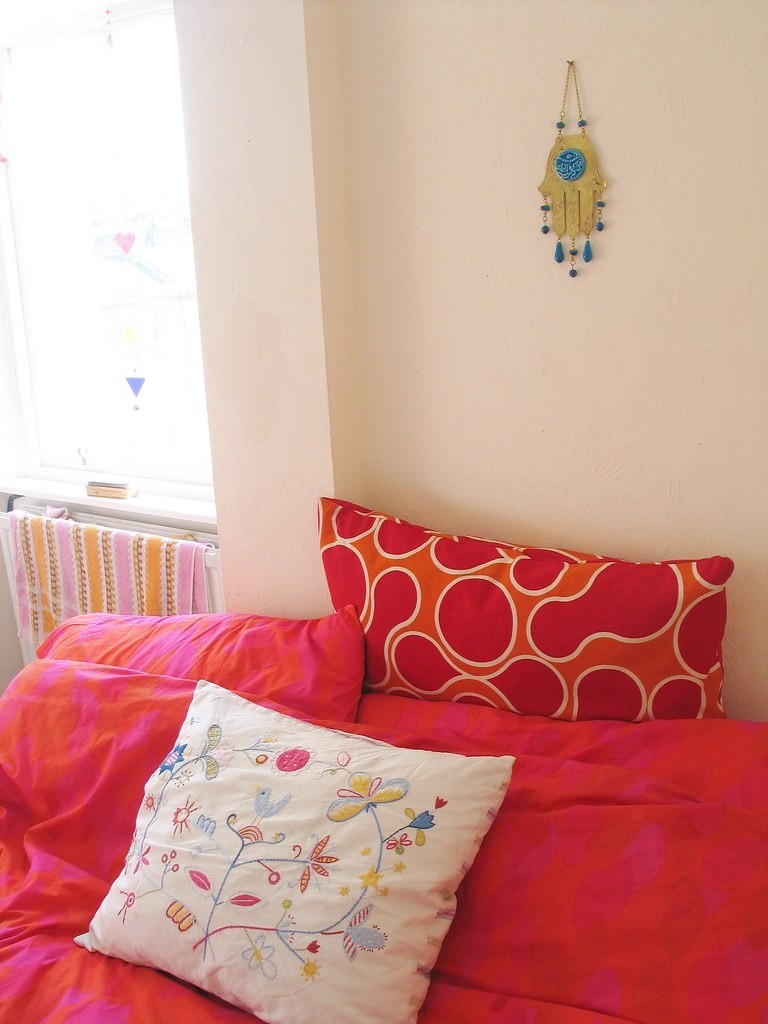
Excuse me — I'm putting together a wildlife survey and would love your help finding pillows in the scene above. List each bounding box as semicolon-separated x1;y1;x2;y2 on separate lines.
36;603;367;724
73;680;516;1024
355;691;768;1024
316;496;734;724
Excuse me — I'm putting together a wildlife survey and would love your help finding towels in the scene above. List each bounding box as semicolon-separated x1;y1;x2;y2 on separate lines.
7;504;214;648
